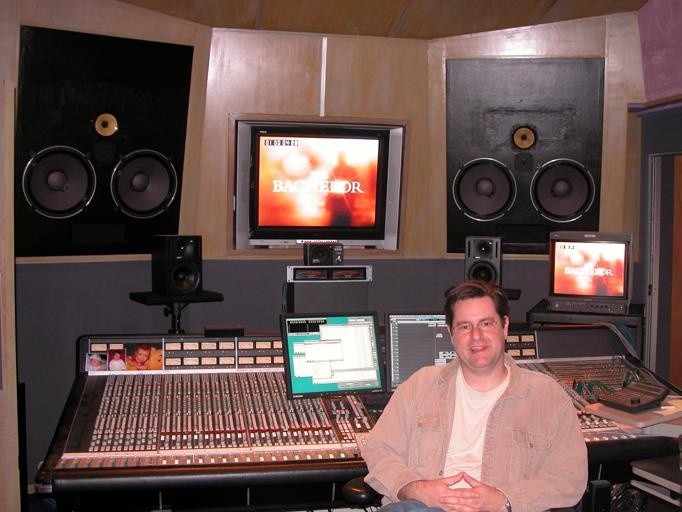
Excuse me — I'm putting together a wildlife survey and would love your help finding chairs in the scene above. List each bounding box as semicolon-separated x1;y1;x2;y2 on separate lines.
344;467;611;512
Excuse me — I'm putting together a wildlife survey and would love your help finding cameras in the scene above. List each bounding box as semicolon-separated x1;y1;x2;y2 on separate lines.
304;242;344;266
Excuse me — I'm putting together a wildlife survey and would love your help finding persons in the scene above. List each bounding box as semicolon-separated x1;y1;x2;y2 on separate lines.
123;343;151;370
361;277;589;512
89;353;105;371
109;351;126;372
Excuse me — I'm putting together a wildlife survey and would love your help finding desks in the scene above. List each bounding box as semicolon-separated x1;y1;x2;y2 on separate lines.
629;452;682;509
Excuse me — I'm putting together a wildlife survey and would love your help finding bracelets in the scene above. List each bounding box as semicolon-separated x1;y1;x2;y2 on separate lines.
506;498;512;512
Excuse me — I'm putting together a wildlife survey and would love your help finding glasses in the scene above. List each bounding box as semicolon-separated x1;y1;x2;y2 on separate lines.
453;319;503;333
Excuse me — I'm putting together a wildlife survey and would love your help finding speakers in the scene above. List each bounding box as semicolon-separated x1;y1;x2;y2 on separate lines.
464;236;503;292
152;234;202;301
446;57;604;255
14;25;194;258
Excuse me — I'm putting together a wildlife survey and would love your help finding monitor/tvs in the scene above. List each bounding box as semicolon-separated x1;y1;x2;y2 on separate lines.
384;310;459;393
546;230;633;316
236;121;404;251
280;310;385;400
